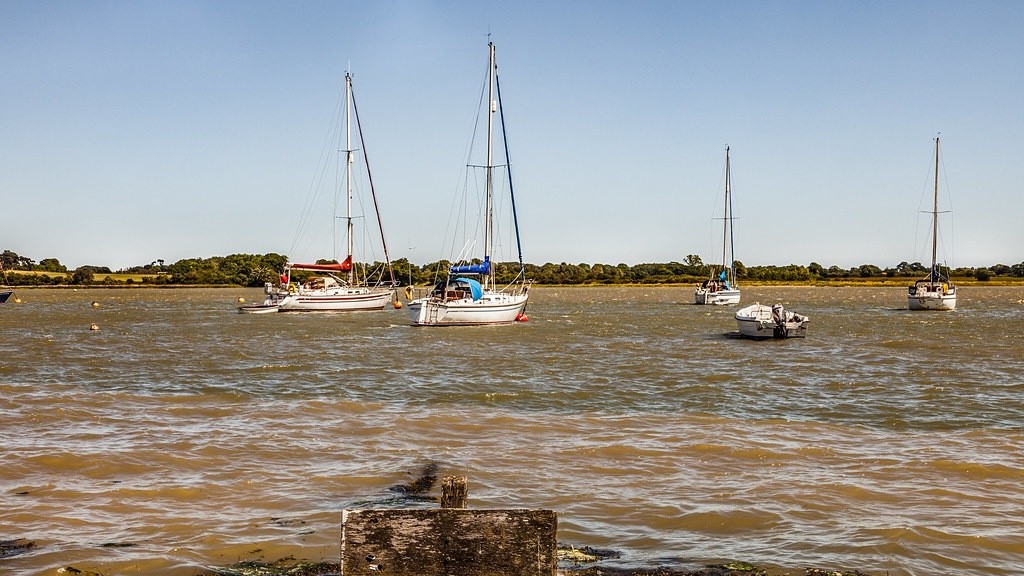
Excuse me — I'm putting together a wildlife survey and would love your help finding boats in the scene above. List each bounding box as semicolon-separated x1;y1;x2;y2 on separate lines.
734;301;811;339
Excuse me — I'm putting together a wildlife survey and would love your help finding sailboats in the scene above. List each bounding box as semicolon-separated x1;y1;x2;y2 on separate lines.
694;143;741;305
406;23;537;326
907;137;957;311
237;57;403;316
